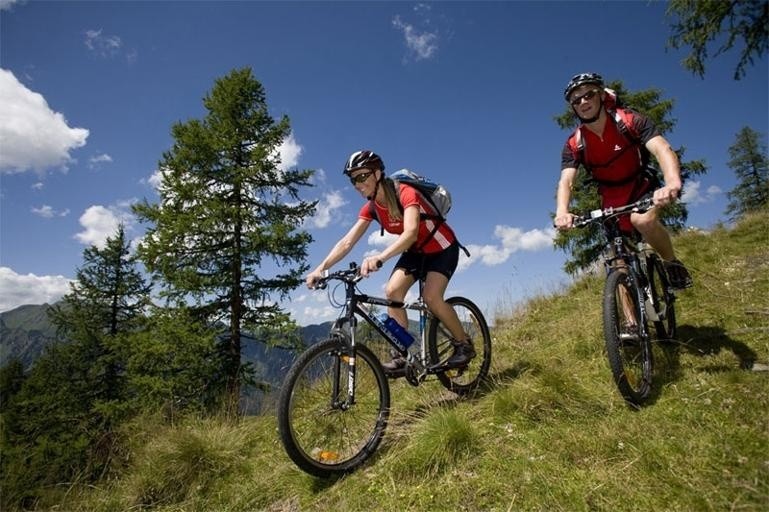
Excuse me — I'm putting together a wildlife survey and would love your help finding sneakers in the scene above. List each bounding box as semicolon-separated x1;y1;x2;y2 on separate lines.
448;333;477;368
662;258;693;289
382;350;408;370
619;324;640;346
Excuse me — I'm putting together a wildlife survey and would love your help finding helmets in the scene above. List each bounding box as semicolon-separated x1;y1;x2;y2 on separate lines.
564;73;603;102
342;150;385;175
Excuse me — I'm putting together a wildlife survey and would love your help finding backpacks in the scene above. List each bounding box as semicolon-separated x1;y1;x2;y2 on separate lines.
369;168;453;222
574;88;637;165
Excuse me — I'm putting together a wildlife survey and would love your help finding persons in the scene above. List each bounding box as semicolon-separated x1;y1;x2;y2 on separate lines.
304;149;479;376
553;70;693;341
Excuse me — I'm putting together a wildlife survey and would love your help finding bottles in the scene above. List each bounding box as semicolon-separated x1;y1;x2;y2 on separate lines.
377;313;415;348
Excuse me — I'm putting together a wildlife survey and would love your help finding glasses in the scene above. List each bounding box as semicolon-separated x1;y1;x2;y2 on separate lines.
350;175;371;183
570;88;604;104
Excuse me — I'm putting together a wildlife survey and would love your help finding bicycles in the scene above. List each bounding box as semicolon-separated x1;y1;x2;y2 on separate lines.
553;190;693;409
277;260;492;480
